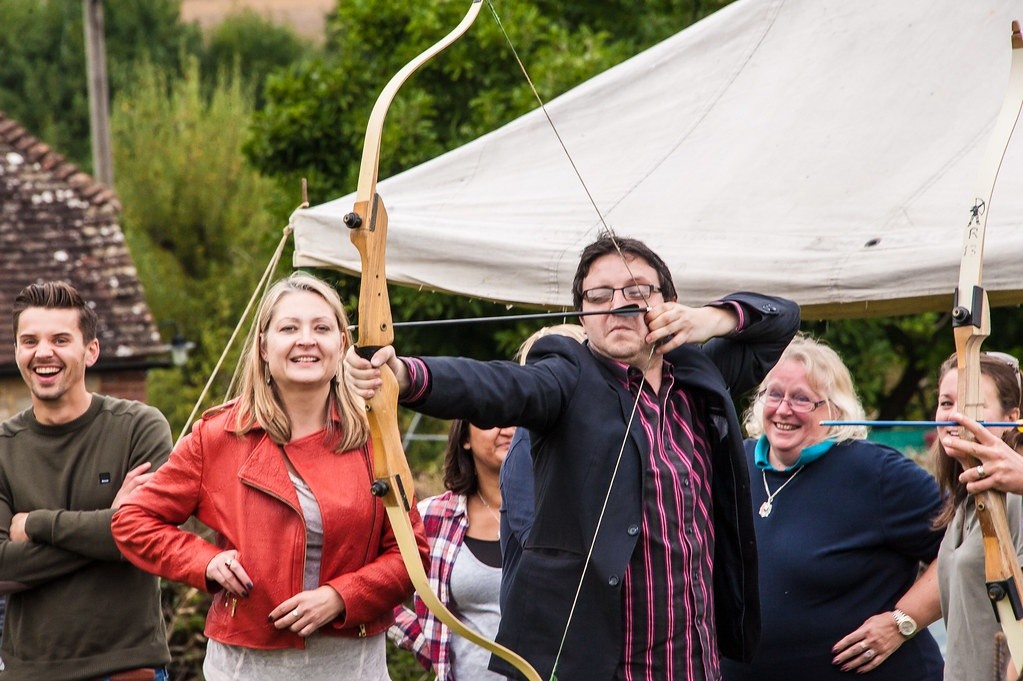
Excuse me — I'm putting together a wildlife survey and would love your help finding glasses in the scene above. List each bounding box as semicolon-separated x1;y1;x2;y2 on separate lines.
763;388;831;413
581;284;661;304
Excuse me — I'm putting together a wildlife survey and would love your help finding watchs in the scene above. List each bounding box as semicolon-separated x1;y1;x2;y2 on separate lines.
893;609;919;639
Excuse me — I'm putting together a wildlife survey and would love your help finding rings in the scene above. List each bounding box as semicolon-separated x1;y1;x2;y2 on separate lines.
227;559;234;568
864;649;874;660
860;641;867;650
977;465;986;479
293;610;299;619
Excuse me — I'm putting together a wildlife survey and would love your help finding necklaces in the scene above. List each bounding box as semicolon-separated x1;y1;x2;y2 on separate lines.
476;491;500;538
759;465;803;518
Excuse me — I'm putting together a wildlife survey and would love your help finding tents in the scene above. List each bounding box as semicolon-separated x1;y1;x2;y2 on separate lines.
291;0;1023;324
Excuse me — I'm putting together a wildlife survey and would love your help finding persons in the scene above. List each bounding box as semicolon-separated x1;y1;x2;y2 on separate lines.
342;231;803;681
742;333;945;680
110;272;431;681
923;352;1023;681
387;421;518;681
0;281;172;681
